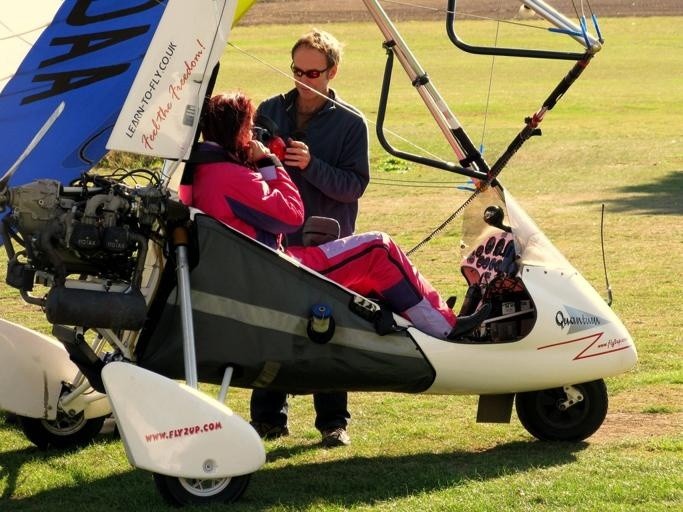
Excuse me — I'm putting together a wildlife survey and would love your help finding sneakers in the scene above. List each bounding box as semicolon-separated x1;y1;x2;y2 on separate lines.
448;304;490;339
250;420;288;438
446;297;455;308
320;428;351;446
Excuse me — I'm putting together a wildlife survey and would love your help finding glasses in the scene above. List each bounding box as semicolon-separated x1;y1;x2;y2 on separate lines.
291;61;332;78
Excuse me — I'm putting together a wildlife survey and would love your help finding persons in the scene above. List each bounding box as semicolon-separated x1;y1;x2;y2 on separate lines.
248;26;370;448
177;91;494;345
